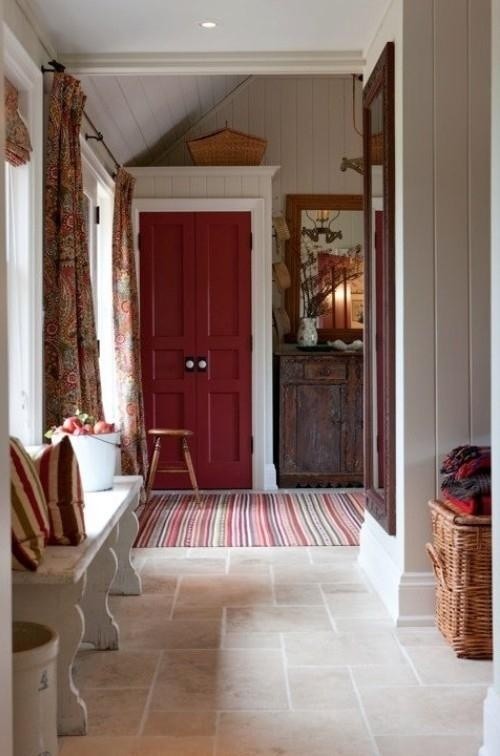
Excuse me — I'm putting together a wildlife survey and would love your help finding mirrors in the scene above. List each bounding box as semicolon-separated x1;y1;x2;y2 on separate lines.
365;44;394;542
285;194;365;344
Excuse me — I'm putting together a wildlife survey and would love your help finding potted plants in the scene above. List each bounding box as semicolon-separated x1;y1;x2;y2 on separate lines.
289;241;363;345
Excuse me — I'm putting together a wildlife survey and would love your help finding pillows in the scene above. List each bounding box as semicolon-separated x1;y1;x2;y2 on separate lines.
9;435;90;573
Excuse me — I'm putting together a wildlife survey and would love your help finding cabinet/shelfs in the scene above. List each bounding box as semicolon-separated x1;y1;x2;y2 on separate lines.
281;356;364;486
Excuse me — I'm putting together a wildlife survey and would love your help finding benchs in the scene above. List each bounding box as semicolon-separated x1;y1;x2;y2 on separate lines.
8;474;145;739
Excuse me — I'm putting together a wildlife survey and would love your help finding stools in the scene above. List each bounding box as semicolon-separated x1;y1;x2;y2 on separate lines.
143;428;199;504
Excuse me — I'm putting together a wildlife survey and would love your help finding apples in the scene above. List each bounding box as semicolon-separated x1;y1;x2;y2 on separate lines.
59;412;114;435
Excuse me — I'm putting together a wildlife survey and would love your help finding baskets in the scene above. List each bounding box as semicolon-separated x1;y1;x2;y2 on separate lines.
424;498;493;664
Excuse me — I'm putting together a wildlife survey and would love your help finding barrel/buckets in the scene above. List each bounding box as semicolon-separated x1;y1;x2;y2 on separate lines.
60;428;122;492
11;620;61;756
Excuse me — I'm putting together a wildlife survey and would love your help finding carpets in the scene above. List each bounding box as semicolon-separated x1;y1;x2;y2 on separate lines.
136;490;365;547
